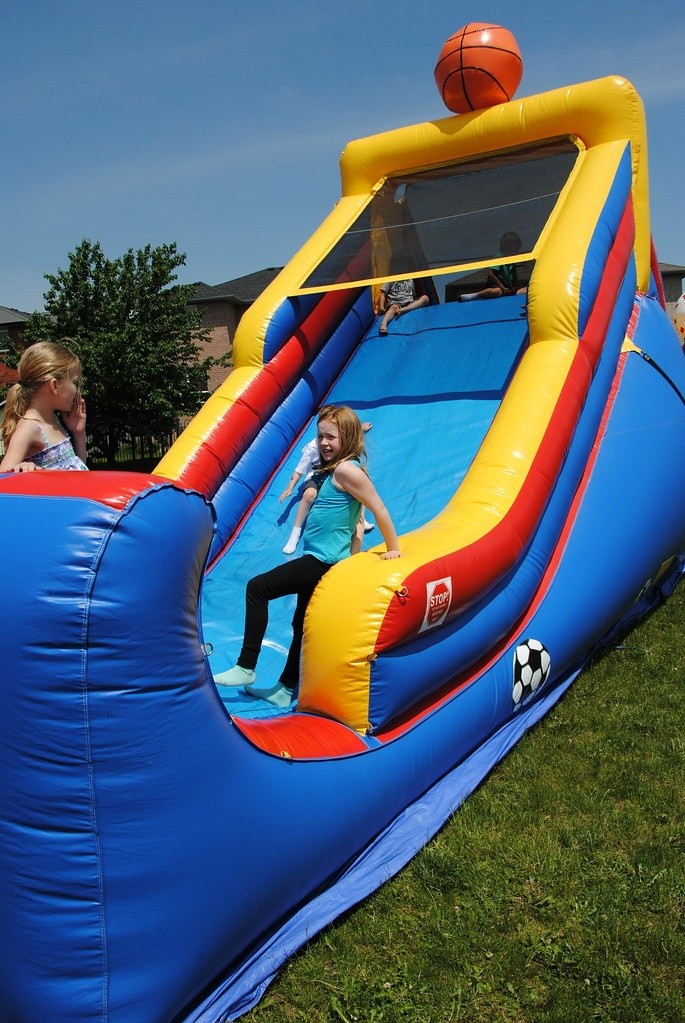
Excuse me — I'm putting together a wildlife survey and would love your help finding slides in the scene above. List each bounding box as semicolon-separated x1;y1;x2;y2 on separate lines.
141;131;640;743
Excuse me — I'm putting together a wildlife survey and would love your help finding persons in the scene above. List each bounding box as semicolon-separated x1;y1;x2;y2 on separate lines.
213;404;401;707
379;254;429;334
0;341;90;473
460;231;527;302
279;422;375;554
